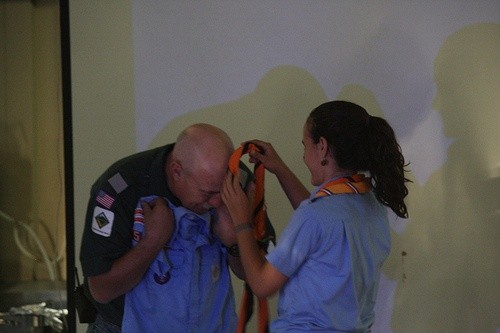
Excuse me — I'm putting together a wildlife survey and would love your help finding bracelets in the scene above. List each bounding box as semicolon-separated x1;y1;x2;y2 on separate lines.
233;223;252;233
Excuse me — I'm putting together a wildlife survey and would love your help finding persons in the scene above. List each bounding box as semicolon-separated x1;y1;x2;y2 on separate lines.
223;100;413;333
79;123;276;333
